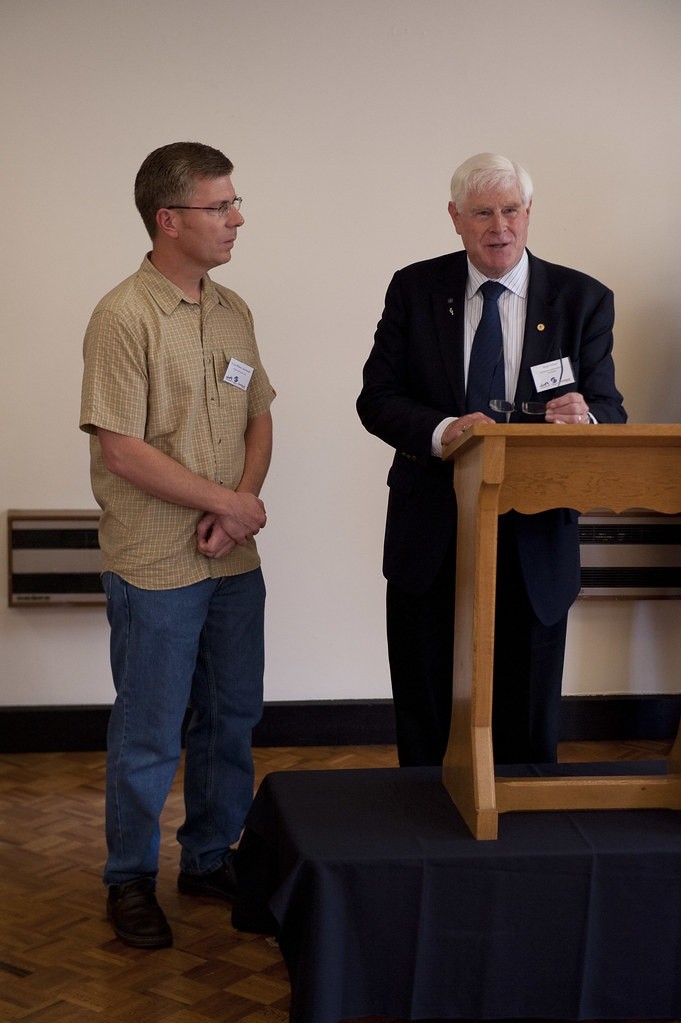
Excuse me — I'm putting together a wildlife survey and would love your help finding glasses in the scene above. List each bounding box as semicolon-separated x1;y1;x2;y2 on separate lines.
487;344;564;415
168;197;242;217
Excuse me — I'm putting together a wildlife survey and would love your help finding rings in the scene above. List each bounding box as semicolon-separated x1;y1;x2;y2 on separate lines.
579;416;581;424
464;425;466;431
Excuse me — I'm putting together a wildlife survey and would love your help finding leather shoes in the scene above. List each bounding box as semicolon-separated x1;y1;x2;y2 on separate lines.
177;848;237;904
105;872;172;946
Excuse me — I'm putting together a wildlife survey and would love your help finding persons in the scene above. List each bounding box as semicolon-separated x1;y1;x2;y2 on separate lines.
79;141;277;949
356;152;628;767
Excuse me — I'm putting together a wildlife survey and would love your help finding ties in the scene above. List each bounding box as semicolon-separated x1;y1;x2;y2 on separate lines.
466;280;510;424
